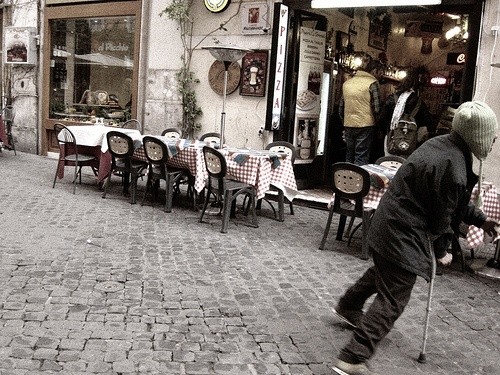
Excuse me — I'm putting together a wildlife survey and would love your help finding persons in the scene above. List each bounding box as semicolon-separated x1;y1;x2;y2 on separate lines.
330;101;499;375
338;60;383;166
373;67;434;164
117;69;133;109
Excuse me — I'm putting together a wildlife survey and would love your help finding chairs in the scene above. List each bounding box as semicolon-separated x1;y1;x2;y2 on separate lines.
318;156;477;276
52;118;296;234
0;106;17;157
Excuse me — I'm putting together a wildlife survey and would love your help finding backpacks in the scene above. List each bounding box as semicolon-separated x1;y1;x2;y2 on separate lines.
387;98;423;156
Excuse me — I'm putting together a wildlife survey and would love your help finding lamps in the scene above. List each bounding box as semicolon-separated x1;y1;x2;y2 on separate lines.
445;26;468;44
200;42;255;65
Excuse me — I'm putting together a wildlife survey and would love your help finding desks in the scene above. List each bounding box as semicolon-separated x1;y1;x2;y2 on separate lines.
200;145;299;222
132;135;228;210
327;163;500;268
73;104;109;108
57;126;141;191
53;112;90;118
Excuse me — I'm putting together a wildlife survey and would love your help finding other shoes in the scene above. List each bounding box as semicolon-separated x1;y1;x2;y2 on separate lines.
332;302;365;328
332;357;377;375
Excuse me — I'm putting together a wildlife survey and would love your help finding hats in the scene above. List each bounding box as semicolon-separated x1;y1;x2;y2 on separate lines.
451;99;498;208
